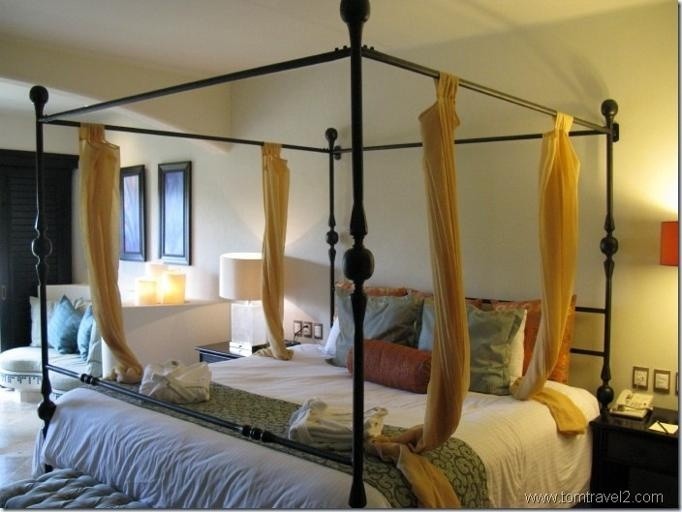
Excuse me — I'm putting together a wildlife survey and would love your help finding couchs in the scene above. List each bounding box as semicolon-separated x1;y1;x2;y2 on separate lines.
28;282;231;381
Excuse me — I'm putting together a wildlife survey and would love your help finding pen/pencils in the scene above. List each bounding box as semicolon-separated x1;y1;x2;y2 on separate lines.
657;420;669;435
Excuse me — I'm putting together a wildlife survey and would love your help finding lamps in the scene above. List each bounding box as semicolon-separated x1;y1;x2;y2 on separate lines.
218;253;262;357
660;220;679;266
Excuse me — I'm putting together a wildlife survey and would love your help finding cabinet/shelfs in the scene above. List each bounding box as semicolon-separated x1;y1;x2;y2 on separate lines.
0;148;78;350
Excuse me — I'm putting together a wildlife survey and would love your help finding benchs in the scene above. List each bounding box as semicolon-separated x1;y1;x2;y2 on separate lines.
1;467;149;511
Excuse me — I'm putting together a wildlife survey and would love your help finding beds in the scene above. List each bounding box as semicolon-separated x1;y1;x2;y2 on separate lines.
30;0;619;510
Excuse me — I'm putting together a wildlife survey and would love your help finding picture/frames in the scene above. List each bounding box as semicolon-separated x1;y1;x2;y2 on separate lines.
121;164;146;263
157;161;191;266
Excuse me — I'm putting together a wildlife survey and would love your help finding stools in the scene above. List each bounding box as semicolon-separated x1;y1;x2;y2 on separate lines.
2;347;42;403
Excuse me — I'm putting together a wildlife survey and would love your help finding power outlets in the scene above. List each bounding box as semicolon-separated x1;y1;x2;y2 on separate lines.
294;321;302;335
632;367;650;389
654;369;670;393
303;321;312;339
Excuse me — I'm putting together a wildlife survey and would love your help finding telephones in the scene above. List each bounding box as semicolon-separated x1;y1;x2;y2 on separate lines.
610;389;654;421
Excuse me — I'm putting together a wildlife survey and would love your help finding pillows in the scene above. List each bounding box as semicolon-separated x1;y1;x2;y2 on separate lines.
492;295;580;383
337;283;408;297
322;291;417;367
418;293;527;395
30;300;57;347
76;304;94;356
55;295;83;354
347;338;432;395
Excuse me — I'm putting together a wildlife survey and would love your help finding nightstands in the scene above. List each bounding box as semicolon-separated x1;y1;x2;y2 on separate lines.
195;341;300;361
588;407;679;510
